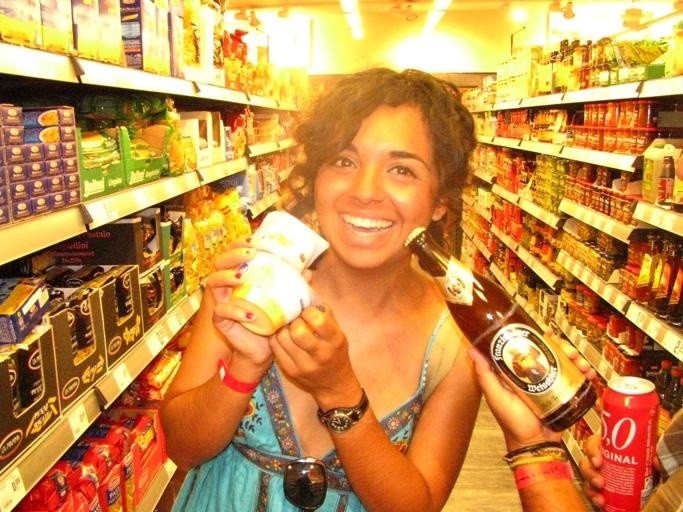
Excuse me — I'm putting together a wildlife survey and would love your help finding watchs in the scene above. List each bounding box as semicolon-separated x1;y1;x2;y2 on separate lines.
316;384;369;432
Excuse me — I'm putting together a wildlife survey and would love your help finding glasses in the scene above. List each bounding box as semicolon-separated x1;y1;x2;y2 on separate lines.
283;455;328;511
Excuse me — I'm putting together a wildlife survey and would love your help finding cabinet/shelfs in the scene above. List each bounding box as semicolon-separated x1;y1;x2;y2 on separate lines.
1;39;317;512
442;40;682;512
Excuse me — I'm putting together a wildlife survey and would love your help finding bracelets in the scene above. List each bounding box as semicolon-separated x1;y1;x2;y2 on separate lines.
501;440;576;490
215;353;261;395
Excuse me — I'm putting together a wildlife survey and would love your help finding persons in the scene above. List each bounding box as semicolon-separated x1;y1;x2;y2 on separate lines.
158;66;484;512
461;335;683;512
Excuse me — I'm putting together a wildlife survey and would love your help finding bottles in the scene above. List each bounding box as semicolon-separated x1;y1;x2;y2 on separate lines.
403;226;598;433
635;143;683;419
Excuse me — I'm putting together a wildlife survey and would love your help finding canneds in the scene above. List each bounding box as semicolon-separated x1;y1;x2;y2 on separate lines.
573;101;683;155
